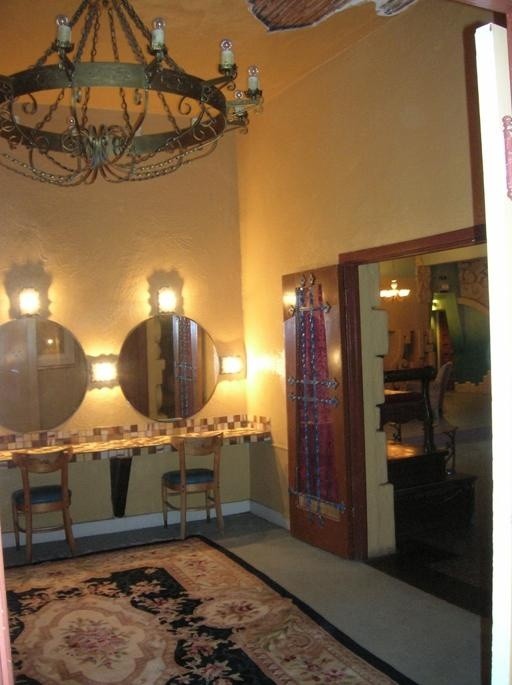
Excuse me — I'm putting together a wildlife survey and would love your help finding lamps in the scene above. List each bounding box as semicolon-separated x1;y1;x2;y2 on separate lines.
1;1;266;186
378;279;411;303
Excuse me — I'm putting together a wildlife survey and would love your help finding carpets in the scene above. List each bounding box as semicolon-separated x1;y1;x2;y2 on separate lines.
3;535;418;685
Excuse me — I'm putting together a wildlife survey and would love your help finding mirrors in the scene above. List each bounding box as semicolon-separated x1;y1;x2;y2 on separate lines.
0;317;90;436
115;313;222;422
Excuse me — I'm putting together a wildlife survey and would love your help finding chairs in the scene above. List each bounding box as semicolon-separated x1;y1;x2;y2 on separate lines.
8;447;80;564
384;366;438;452
161;433;225;540
